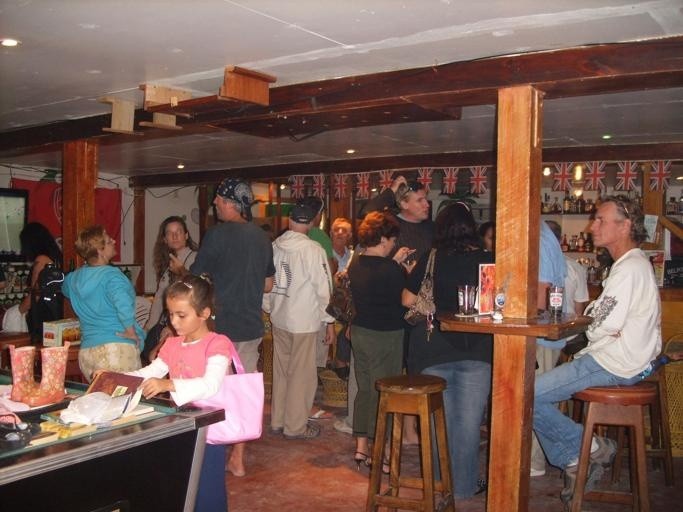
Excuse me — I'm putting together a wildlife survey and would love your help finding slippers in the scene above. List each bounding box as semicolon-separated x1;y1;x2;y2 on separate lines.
308;409;337;421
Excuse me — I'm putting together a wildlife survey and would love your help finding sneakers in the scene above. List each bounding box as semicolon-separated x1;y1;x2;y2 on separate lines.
530;465;546;477
475;479;488;495
283;423;320;440
333;420;353;434
588;432;618;480
559;457;605;502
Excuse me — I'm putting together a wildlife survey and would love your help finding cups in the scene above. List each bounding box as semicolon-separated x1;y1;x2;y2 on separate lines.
547;288;565;323
458;286;475;316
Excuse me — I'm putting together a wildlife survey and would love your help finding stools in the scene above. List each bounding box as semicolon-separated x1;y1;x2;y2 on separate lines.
607;364;674;487
565;378;658;511
365;372;454;512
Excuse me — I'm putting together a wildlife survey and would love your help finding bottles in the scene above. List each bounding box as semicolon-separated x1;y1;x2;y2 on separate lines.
666;197;679;215
576;196;585;214
584;233;593;252
561;234;568;252
563;190;571;213
577;232;584;251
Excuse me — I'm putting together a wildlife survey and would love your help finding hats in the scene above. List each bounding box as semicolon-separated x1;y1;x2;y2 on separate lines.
216;177;255;207
288;204;315;225
303;196;328;214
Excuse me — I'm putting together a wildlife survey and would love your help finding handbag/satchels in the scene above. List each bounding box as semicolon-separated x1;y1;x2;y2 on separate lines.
325;265;357;322
25;263;66;297
403;247;438;343
139;249;195;365
191;334;265;445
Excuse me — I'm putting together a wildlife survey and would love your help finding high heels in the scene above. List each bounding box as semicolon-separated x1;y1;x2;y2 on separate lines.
353;451;371;471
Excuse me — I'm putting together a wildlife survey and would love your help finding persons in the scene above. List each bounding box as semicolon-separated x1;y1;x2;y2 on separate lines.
480;221;496;252
262;205;338;438
347;212;418;476
142;217;199;334
334;199;416;435
531;217;567;475
401;201;494;502
168;177;276;477
305;194;336;420
533;196;662;503
328;218;353;381
61;226;147;383
358;176;435;447
92;273;231;512
143;216;199;333
18;222;63;345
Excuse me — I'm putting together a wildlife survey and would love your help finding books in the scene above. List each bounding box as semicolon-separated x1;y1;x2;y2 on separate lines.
40;422;99;439
545;221;588;345
40;409;136;428
28;430;59;448
83;370;146;419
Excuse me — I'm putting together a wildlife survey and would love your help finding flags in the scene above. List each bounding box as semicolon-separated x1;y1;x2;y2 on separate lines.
378;170;393;194
334;173;350;201
417;167;434;192
313;174;327;198
551;162;577;192
354;172;369;200
442;166;459;194
649;159;672;191
582;160;607;192
614;160;639;191
468;165;488;194
290;175;305;199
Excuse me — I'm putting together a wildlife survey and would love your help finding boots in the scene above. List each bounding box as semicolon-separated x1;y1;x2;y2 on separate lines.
8;344;40;402
27;339;72;407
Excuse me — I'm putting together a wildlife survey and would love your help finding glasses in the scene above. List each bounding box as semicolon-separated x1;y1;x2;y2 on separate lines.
398;181;423;200
